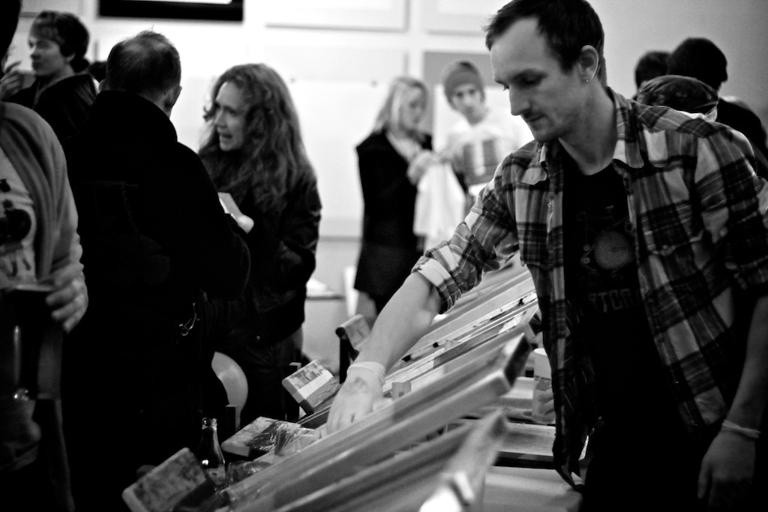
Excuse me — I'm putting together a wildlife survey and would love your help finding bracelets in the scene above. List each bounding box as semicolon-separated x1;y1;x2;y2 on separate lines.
719;421;758;439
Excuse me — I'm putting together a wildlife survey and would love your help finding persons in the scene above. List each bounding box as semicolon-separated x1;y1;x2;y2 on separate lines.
1;0;89;511
669;38;766;166
440;60;518;207
634;48;678;91
634;75;718;120
322;1;767;510
355;76;445;318
2;12;109;128
199;63;325;423
669;39;766;166
57;32;251;510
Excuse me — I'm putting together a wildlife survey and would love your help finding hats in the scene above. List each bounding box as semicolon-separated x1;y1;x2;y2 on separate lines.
30;10;89;58
442;59;485;109
637;39;729;112
